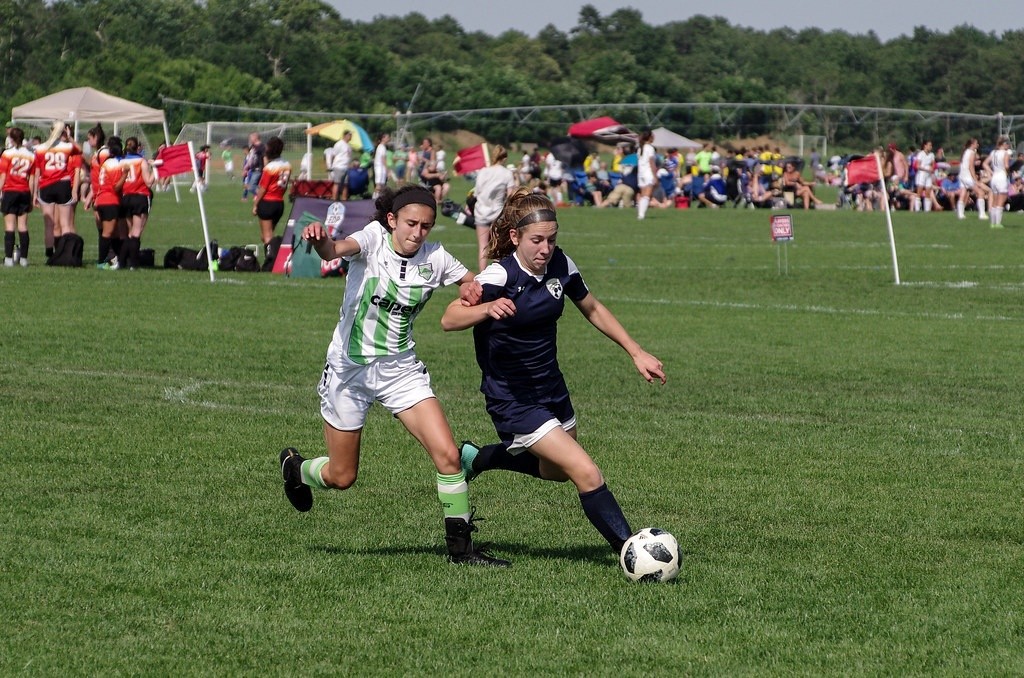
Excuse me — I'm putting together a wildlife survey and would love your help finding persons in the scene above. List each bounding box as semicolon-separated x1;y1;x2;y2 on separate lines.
878;143;1024;212
279;179;513;570
637;128;658;220
440;187;667;554
0;118;177;270
330;128;353;200
472;145;513;273
914;140;937;212
504;145;638;205
983;137;1010;227
373;132;391;199
653;143;822;210
253;135;293;260
955;137;990;219
808;147;885;211
298;137;452;203
187;133;269;200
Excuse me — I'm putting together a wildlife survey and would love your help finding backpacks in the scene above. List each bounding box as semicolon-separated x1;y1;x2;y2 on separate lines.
140;241;259;274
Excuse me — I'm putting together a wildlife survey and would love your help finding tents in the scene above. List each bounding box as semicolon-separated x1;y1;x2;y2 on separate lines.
12;86;183;203
271;194;380;274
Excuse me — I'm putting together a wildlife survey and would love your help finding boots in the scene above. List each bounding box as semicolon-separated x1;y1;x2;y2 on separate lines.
915;198;921;212
924;198;932;212
444;506;512;570
991;208;995;229
280;447;313;512
638;197;650;218
995;207;1004;228
957;202;966;219
977;199;990;220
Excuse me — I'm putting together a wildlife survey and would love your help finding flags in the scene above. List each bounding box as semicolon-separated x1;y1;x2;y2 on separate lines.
452;143;491;176
847;154;880;185
153;142;194;179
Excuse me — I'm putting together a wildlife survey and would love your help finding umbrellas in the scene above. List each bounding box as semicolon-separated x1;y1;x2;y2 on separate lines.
651;126;703;148
304;119;373;152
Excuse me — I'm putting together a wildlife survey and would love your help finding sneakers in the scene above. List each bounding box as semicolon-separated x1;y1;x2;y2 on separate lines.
459;441;480;485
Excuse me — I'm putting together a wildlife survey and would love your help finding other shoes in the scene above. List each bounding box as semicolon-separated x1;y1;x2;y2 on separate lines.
20;257;28;267
42;253;139;272
4;257;13;267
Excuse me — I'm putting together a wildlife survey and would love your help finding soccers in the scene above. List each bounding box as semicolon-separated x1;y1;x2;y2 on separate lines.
619;526;684;584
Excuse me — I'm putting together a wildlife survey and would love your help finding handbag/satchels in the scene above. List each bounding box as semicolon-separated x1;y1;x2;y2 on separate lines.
46;233;84;268
261;235;284;273
